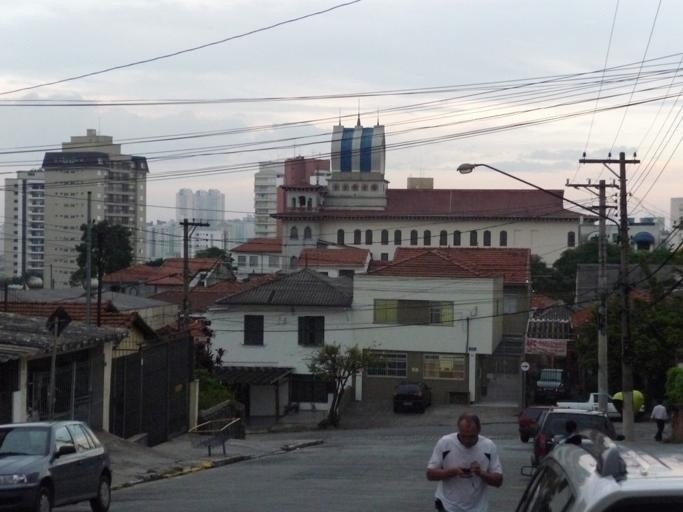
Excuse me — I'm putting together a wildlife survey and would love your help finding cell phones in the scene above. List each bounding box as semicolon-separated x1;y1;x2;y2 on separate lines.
462;466;471;474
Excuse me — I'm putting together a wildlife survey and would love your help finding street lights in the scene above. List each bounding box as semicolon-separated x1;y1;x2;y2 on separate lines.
457;163;634;439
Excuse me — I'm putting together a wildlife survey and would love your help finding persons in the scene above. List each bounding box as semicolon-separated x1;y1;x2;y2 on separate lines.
424;411;503;512
649;400;669;442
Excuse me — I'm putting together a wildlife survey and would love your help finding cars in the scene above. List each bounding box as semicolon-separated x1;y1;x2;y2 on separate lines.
0;420;112;511
515;369;683;512
392;380;432;414
